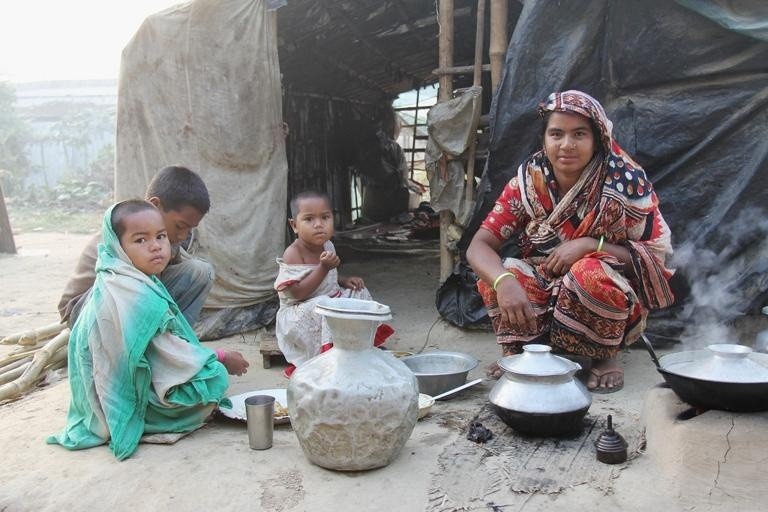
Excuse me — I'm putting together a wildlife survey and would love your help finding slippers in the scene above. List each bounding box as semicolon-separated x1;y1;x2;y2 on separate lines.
587;366;625;395
485;361;502;381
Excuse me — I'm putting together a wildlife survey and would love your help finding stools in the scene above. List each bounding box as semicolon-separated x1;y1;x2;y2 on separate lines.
259;340;284;369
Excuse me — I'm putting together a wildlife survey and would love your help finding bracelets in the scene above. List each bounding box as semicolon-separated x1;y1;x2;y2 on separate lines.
597;235;605;252
216;348;227;362
494;273;516;292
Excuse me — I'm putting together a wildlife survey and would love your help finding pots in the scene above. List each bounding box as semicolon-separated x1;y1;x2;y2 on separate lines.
488;344;593;438
636;333;768;415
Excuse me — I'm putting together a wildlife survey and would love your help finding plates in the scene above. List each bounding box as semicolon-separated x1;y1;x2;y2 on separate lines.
418;392;435;421
219;388;288;427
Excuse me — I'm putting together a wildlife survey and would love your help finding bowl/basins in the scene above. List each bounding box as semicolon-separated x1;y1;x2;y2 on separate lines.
399;351;476;401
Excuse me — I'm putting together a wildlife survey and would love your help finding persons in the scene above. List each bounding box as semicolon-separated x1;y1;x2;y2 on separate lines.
58;165;215;337
65;199;249;423
465;90;679;395
360;121;409;224
273;188;395;379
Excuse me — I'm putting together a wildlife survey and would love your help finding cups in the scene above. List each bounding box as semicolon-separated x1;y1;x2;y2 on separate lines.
244;395;277;451
